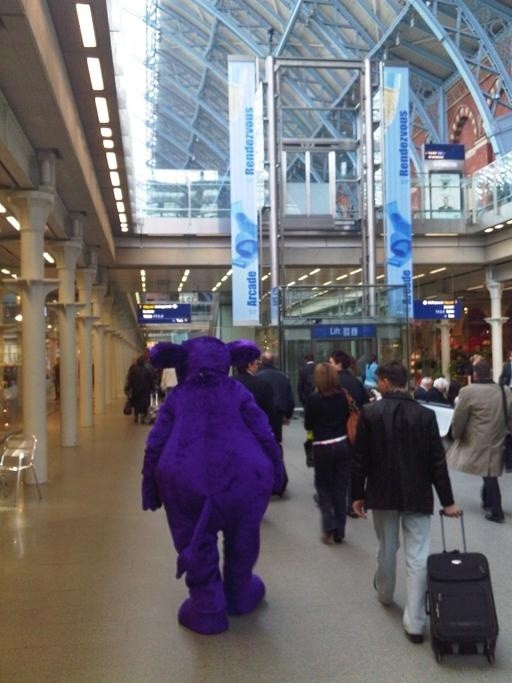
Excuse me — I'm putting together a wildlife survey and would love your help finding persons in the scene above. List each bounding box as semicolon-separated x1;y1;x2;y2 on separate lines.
414;346;437;367
297;349;378;546
351;360;463;644
214;185;231;217
477;177;511;211
438;196;453;211
413;377;451;404
443;345;512;523
124;355;159;424
51;357;61;399
231;351;296;499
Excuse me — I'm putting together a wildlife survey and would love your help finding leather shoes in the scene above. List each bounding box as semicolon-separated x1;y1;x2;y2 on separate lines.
485;514;501;523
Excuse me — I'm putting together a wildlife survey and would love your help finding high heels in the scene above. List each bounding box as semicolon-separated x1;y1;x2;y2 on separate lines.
320;528;337;544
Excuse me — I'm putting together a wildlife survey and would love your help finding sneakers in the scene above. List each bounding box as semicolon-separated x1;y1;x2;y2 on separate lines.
405;630;424;643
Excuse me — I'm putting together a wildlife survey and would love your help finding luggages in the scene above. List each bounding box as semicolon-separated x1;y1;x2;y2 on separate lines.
143;392;160;425
425;508;499;664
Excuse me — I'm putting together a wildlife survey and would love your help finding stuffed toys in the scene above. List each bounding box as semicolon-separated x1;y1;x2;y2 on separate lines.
142;336;286;634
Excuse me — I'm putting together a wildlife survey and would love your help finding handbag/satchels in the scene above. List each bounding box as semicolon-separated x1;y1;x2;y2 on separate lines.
304;431;314;466
124;399;131;415
347;399;361;446
504;434;511;472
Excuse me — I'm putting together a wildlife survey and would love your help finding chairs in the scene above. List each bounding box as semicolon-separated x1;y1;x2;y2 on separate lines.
1;434;41;509
416;398;455;440
